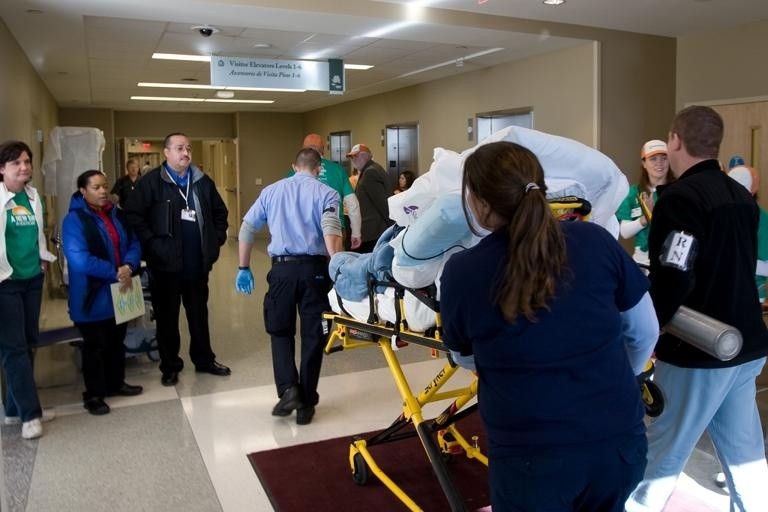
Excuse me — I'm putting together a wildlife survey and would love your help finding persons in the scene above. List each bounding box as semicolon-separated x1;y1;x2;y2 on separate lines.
623;105;768;512
61;169;143;416
124;133;230;386
346;143;394;253
302;133;362;250
235;148;343;425
727;157;759;199
0;139;58;440
394;171;416;194
343;168;362;217
618;140;670;278
110;159;141;208
441;141;660;511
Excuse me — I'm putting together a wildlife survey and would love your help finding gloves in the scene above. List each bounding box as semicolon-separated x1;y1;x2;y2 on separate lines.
236;269;254;295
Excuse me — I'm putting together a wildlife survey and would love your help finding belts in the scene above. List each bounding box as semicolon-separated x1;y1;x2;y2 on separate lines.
271;256;329;263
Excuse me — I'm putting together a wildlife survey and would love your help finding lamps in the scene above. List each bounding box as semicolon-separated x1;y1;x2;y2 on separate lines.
129;51;375;106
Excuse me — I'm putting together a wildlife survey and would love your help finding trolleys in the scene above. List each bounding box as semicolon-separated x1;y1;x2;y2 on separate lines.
321;202;667;512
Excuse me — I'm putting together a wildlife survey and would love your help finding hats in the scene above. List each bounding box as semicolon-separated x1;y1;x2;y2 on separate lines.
641;140;669;161
346;144;371;158
304;134;324;149
727;165;759;194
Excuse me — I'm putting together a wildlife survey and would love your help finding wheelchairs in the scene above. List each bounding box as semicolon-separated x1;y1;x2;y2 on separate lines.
50;235;160;362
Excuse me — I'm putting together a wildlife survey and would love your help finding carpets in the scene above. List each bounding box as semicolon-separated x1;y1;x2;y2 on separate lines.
246;402;491;512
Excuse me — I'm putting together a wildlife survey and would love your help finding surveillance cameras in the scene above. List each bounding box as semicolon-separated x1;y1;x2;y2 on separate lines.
190;26;221;38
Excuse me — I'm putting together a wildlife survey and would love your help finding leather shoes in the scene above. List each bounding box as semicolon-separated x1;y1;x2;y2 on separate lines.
161;369;179;386
297;407;314;425
272;388;302;417
194;361;231;376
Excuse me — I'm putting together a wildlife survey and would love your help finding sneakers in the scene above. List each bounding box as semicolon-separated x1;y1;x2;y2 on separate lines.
21;418;44;440
84;400;110;416
4;408;57;425
106;381;143;397
